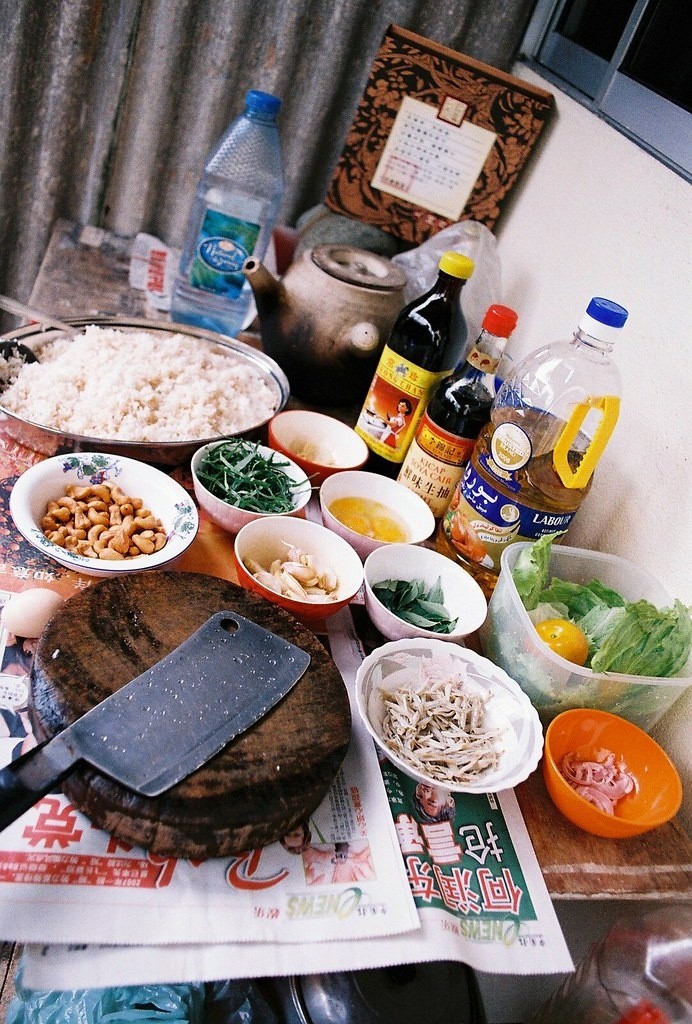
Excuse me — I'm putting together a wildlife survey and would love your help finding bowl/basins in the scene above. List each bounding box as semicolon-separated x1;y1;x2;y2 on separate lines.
269;410;369;487
480;541;691;732
234;516;364;624
320;471;435;565
363;544;488;640
191;440;312;533
0;316;289;476
9;452;199;577
355;637;544;793
543;709;683;837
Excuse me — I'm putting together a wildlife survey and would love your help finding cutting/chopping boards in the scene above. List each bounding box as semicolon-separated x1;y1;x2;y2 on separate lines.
27;571;352;857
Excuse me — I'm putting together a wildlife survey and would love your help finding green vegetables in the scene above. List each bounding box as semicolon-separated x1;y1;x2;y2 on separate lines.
196;435;321;515
374;576;459;634
489;530;691;729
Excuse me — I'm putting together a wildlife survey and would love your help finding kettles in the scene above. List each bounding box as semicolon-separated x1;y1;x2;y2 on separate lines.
242;243;408;430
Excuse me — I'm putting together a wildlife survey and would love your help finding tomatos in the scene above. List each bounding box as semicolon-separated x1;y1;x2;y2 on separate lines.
525;619;588;671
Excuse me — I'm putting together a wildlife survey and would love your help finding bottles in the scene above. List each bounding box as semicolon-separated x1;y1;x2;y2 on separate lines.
395;304;518;540
354;251;474;481
420;297;628;607
170;89;283;339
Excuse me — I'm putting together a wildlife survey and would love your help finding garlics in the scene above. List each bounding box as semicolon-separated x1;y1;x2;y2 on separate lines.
244;544;338;604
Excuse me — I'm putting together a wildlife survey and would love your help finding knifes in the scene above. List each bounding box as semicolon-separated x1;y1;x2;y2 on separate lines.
0;611;311;833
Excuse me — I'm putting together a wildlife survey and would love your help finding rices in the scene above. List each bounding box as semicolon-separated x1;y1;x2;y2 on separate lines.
0;324;278;442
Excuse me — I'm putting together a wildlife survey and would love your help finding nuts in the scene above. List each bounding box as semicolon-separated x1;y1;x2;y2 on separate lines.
42;483;166;559
